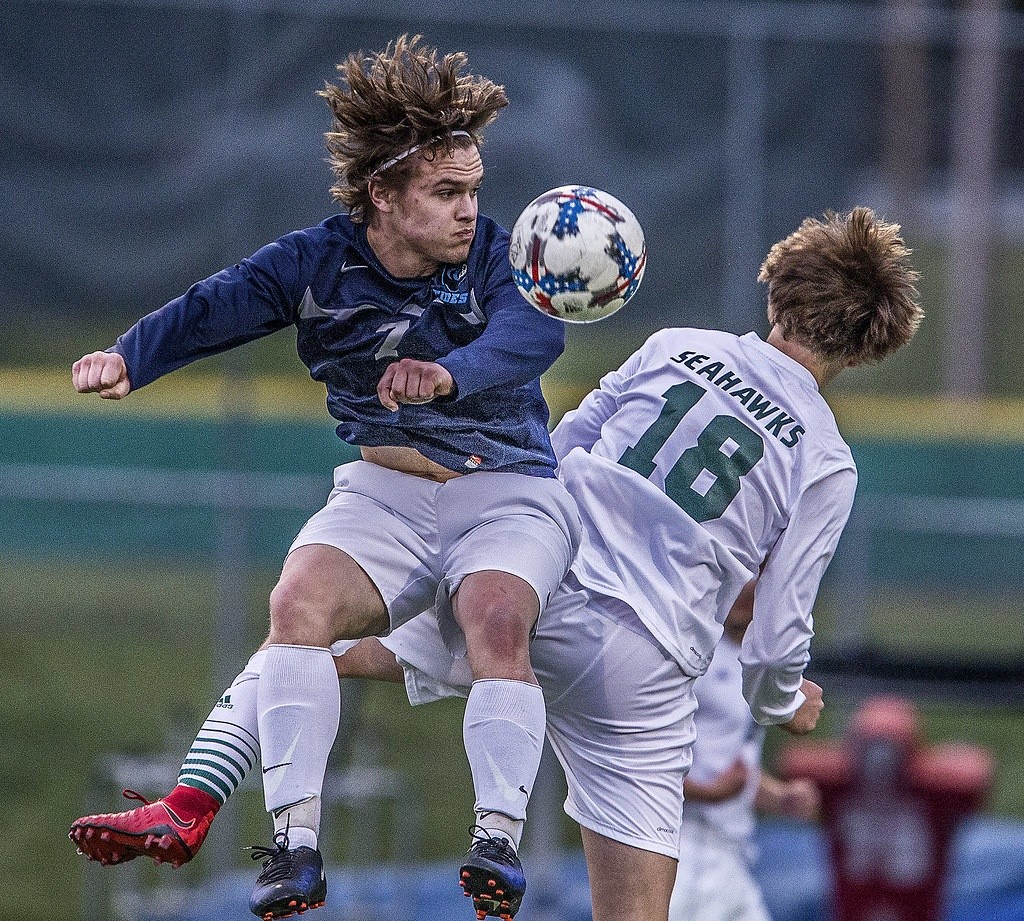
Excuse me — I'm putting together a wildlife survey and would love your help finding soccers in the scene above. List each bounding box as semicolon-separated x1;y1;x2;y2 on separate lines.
509;185;646;324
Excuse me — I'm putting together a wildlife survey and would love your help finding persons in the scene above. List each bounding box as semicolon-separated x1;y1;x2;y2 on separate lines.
772;697;993;921
69;202;928;921
71;33;582;921
669;561;816;921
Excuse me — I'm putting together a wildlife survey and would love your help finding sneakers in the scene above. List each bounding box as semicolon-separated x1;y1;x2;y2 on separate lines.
68;784;220;870
458;823;527;921
239;814;327;921
